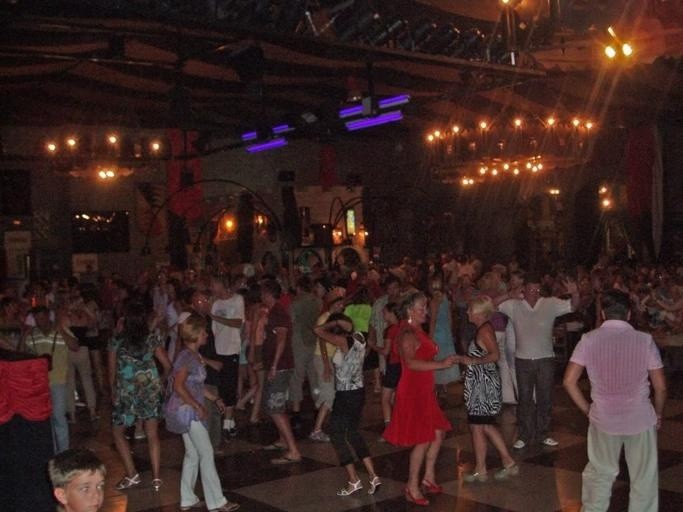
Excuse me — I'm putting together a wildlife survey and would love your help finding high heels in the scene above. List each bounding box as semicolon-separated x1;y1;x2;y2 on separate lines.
420;478;448;496
403;484;431;506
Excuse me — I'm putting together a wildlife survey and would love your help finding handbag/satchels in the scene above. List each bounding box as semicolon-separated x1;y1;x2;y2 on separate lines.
164;409;192;434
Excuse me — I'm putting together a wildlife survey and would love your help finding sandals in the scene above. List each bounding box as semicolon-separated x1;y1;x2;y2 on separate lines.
336;479;364;496
115;473;142;491
367;476;383;495
151;478;164;492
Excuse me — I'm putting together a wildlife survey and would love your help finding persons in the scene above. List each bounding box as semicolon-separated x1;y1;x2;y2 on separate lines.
0;257;682;510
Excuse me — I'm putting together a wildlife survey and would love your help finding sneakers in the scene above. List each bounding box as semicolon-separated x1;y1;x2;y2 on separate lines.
538;438;559;447
311;430;329;444
462;468;488;483
512;440;529;450
493;461;520;480
216;426;232;444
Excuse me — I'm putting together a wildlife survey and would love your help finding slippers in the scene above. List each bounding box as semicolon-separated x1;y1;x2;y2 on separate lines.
270;455;302;465
263;443;288;451
